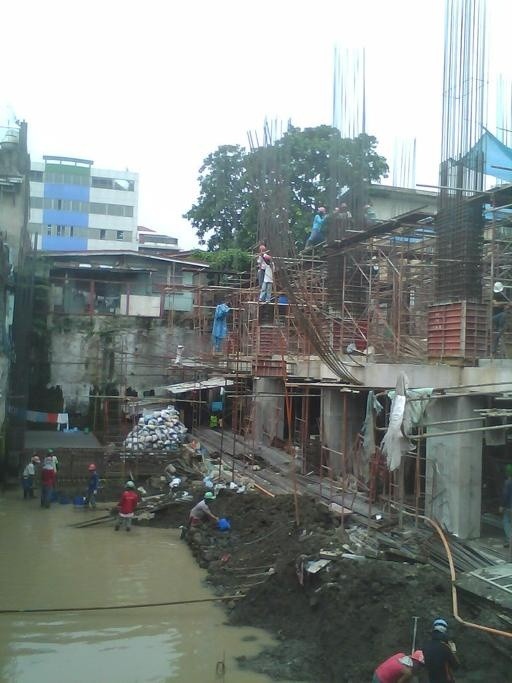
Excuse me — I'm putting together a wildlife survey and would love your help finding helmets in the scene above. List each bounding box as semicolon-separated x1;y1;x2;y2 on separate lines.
88;464;96;471
411;650;426;665
126;480;135;489
493;281;503;293
433;619;449;628
31;448;54;469
318;203;371;212
259;245;266;251
204;491;216;500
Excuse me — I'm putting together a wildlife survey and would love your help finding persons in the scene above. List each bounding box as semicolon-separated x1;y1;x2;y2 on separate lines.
114;480;140;531
257;245;268;289
259;253;276;303
41;457;54;507
180;491;220;539
422;618;461;683
498;463;512;548
211;299;230;357
372;649;425;683
22;456;41;499
47;448;60;474
304;203;378;248
84;463;99;506
492;282;512;355
